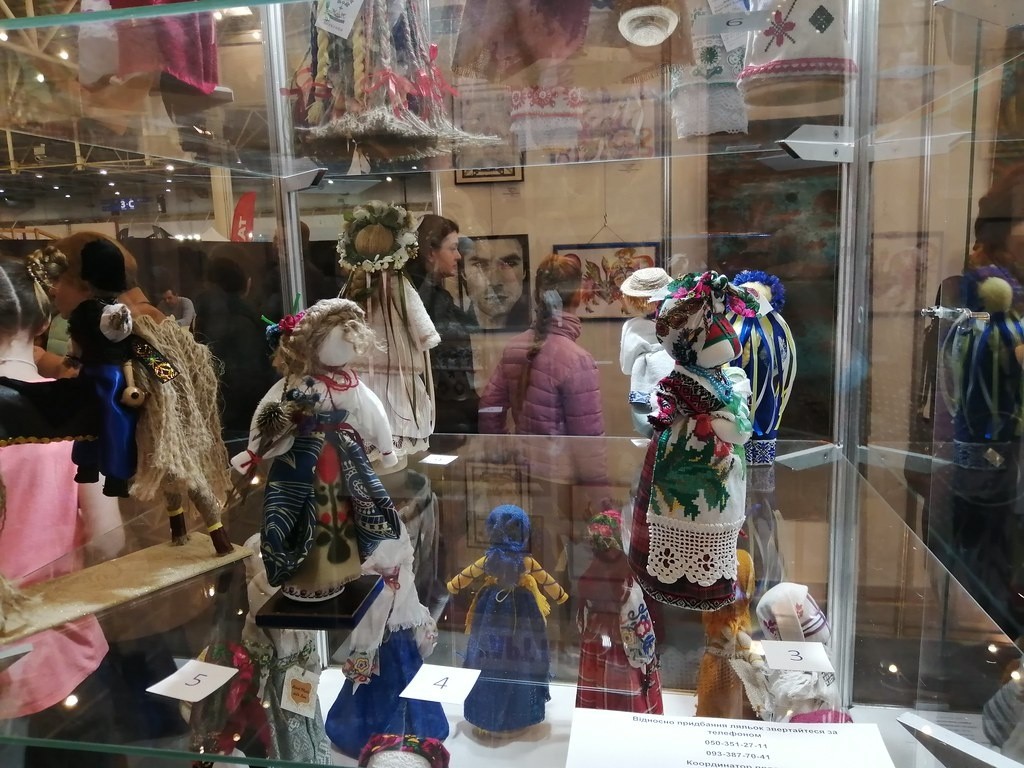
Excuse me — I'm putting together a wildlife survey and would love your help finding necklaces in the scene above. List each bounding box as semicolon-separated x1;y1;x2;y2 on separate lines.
0;356;37;373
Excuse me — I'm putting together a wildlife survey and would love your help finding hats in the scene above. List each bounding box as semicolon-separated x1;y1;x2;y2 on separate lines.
42;230;137;273
619;266;674;298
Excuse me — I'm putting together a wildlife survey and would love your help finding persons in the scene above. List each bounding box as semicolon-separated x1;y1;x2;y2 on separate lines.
409;211;479;434
460;241;525;327
153;285;197;328
479;255;607;598
1;260;126;767
620;266;673;436
33;231;226;739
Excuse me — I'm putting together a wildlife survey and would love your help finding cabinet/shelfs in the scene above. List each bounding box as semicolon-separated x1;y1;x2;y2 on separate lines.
0;0;1024;768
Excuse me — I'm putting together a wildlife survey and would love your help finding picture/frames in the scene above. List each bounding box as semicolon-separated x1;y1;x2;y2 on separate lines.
550;241;661;323
455;234;529;334
450;82;525;186
